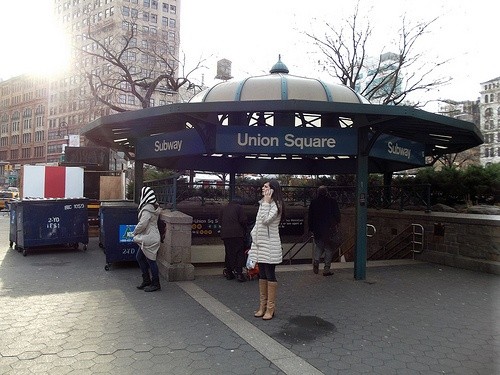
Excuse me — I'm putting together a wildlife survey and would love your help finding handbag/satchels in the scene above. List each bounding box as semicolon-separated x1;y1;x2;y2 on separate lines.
157;215;166;243
327;228;343;250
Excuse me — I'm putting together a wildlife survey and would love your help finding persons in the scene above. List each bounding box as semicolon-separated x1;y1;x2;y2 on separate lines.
249;180;283;319
308;186;340;276
219;197;248;282
132;187;162;292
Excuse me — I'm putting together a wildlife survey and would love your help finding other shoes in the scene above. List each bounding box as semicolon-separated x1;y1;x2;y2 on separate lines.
323;272;334;276
223;266;246;282
312;262;320;274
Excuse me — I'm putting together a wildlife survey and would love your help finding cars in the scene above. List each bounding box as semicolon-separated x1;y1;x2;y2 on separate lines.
0;184;20;211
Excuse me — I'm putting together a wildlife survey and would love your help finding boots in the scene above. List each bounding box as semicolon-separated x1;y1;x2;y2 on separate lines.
136;274;160;292
254;279;277;319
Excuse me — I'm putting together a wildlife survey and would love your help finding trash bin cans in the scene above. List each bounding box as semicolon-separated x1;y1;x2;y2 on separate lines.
98;201;140;271
4;197;90;256
